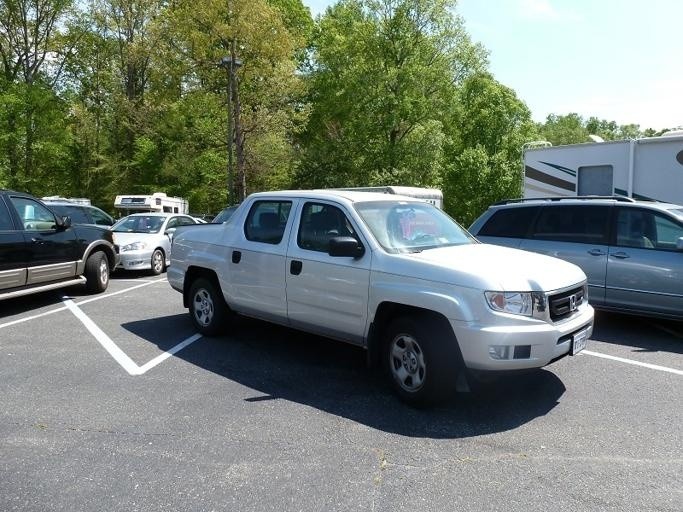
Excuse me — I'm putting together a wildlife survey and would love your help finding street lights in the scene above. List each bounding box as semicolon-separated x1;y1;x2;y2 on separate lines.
214;56;243;206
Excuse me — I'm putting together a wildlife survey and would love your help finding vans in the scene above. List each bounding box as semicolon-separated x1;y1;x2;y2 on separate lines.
460;190;683;332
113;192;189;225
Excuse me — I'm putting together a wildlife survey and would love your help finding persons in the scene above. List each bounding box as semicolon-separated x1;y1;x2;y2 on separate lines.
325;211;363;248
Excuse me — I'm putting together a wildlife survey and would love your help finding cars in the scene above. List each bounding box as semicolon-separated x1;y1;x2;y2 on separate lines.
169;189;596;403
108;212;201;276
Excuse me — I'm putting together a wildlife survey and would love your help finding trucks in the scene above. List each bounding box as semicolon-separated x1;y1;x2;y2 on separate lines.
521;132;682;210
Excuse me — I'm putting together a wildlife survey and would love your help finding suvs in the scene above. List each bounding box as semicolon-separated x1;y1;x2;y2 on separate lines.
0;189;117;303
22;202;119;231
